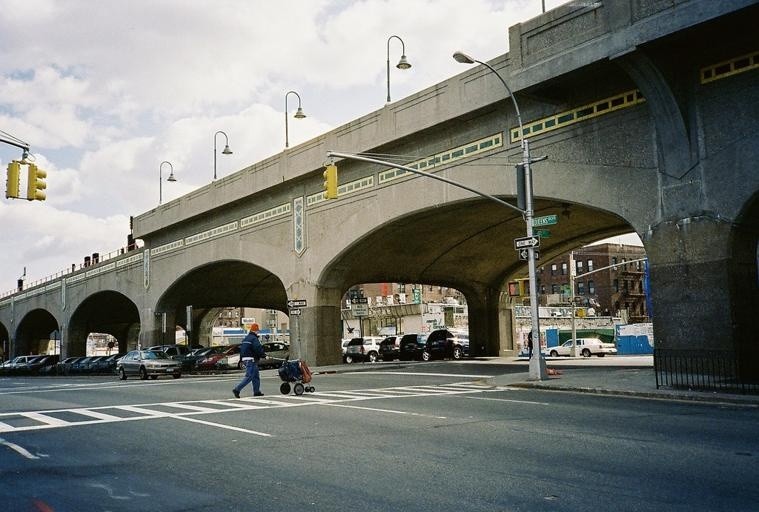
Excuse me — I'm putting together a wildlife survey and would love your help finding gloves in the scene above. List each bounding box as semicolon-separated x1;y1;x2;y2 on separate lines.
265;356;269;360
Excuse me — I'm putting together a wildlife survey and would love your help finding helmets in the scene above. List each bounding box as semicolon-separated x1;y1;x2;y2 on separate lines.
251;324;259;331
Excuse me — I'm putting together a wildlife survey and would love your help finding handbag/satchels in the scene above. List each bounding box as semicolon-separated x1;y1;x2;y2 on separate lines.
278;361;300;381
301;360;312;384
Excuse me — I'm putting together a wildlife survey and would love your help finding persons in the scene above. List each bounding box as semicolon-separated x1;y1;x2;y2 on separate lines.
232;324;270;399
528;329;533;359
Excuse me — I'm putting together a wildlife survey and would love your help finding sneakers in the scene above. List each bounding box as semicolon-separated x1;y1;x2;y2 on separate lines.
233;388;240;398
254;392;264;396
